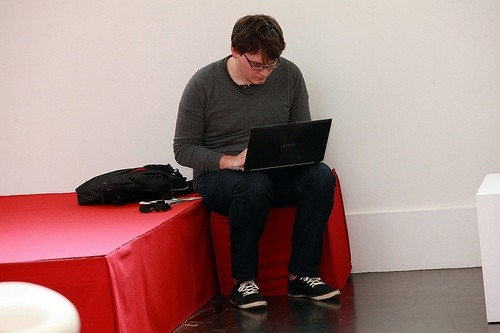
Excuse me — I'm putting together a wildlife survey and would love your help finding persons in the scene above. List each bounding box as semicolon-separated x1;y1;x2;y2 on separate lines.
175;12;342;312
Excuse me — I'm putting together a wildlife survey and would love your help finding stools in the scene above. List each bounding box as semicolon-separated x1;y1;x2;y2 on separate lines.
475;175;500;323
210;169;352;296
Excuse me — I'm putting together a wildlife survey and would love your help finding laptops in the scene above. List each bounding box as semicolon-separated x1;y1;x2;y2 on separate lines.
225;119;333;174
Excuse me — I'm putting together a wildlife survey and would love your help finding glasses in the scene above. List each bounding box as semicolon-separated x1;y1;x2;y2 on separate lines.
242;52;281;70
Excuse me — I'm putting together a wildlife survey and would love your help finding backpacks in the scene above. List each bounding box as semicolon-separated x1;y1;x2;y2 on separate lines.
75;163;195;206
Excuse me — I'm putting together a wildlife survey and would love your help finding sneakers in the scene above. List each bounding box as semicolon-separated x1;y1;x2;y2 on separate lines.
288;275;340;300
230;279;267;309
